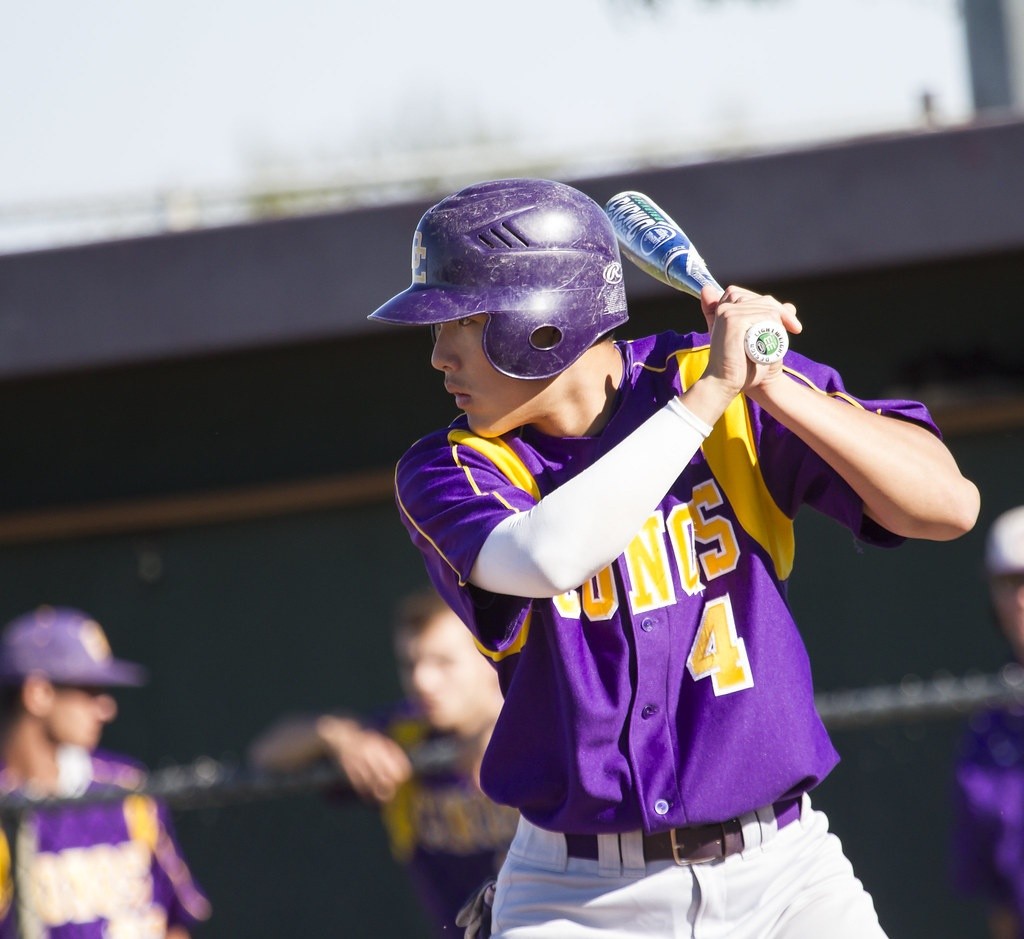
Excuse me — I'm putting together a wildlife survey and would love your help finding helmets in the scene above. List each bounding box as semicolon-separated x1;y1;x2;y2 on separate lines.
367;178;630;380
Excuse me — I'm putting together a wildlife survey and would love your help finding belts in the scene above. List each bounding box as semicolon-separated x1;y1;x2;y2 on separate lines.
566;817;744;866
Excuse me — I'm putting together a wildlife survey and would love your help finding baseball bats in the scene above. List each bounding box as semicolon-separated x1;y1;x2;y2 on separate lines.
604;189;790;365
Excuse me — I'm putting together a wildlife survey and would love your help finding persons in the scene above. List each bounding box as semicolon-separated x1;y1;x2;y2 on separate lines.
367;178;980;939
0;607;213;939
946;511;1024;939
250;584;520;939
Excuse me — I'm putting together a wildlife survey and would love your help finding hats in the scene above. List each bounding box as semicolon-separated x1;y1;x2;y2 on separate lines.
986;506;1024;572
1;605;150;688
